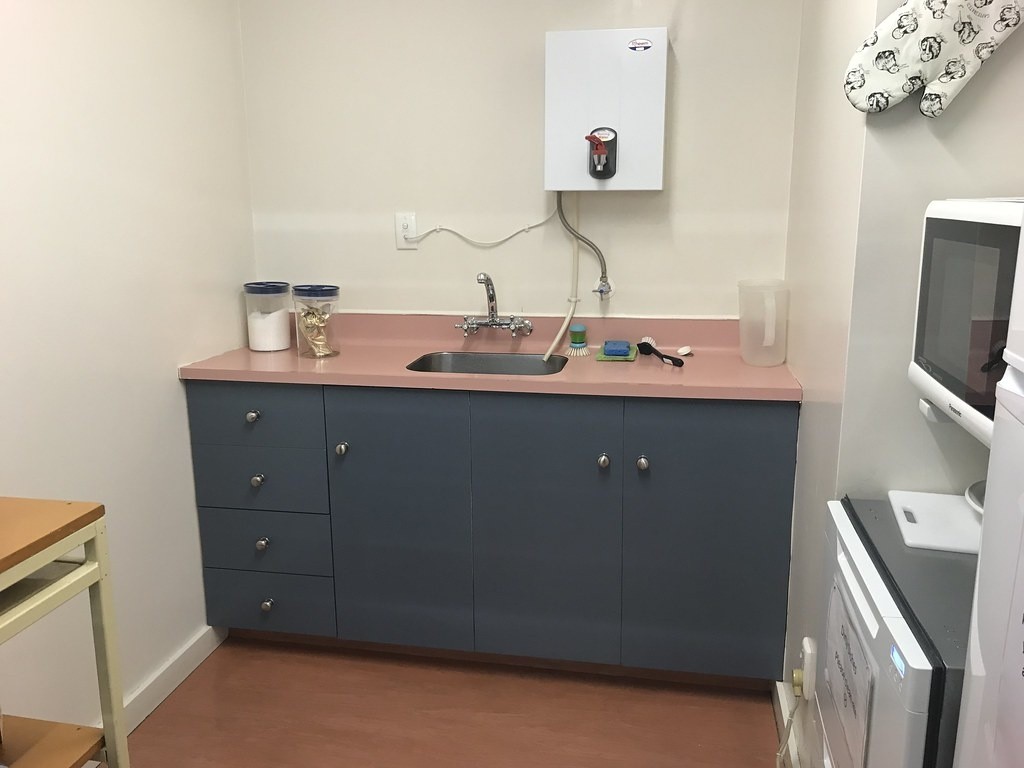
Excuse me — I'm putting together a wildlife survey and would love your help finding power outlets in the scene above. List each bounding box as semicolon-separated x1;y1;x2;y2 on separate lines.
395;212;418;250
800;637;818;701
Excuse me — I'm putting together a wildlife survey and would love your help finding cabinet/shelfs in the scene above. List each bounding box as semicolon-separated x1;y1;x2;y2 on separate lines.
324;386;475;662
184;379;337;646
469;390;801;693
0;496;130;768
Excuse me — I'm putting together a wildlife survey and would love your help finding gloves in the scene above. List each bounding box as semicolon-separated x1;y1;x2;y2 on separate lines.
843;0;1024;119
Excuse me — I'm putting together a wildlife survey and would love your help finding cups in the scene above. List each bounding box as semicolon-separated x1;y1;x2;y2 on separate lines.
588;127;618;179
243;280;291;352
292;284;341;360
736;277;789;367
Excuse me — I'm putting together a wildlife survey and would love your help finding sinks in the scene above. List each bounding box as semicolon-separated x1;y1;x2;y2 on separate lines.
407;350;569;376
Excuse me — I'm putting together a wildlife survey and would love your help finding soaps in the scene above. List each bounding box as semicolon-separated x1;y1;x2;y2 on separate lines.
604;341;630;356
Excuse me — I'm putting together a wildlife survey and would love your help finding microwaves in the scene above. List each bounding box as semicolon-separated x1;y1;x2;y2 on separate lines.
903;194;1024;451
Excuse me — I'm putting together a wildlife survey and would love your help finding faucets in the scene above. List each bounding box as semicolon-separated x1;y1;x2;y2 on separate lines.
453;272;533;342
